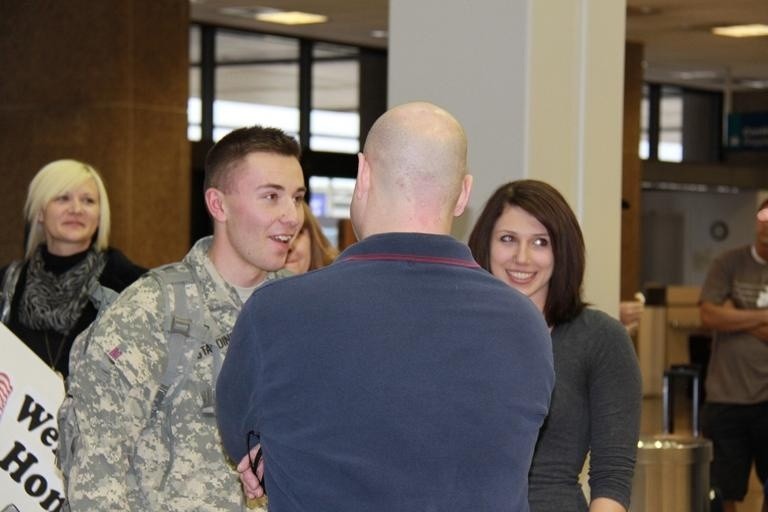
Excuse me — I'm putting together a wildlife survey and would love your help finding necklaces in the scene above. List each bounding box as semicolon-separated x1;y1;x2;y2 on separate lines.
37;280;85;370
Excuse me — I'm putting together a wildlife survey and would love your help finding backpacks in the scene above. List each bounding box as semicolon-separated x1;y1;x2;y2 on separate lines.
54;261;206;512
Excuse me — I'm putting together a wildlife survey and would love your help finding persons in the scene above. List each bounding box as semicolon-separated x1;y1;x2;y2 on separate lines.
466;180;643;509
699;190;768;511
58;125;310;512
216;100;559;508
0;159;149;377
284;198;340;276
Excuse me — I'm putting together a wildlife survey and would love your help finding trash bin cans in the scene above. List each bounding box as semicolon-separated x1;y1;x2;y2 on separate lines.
628;435;714;512
